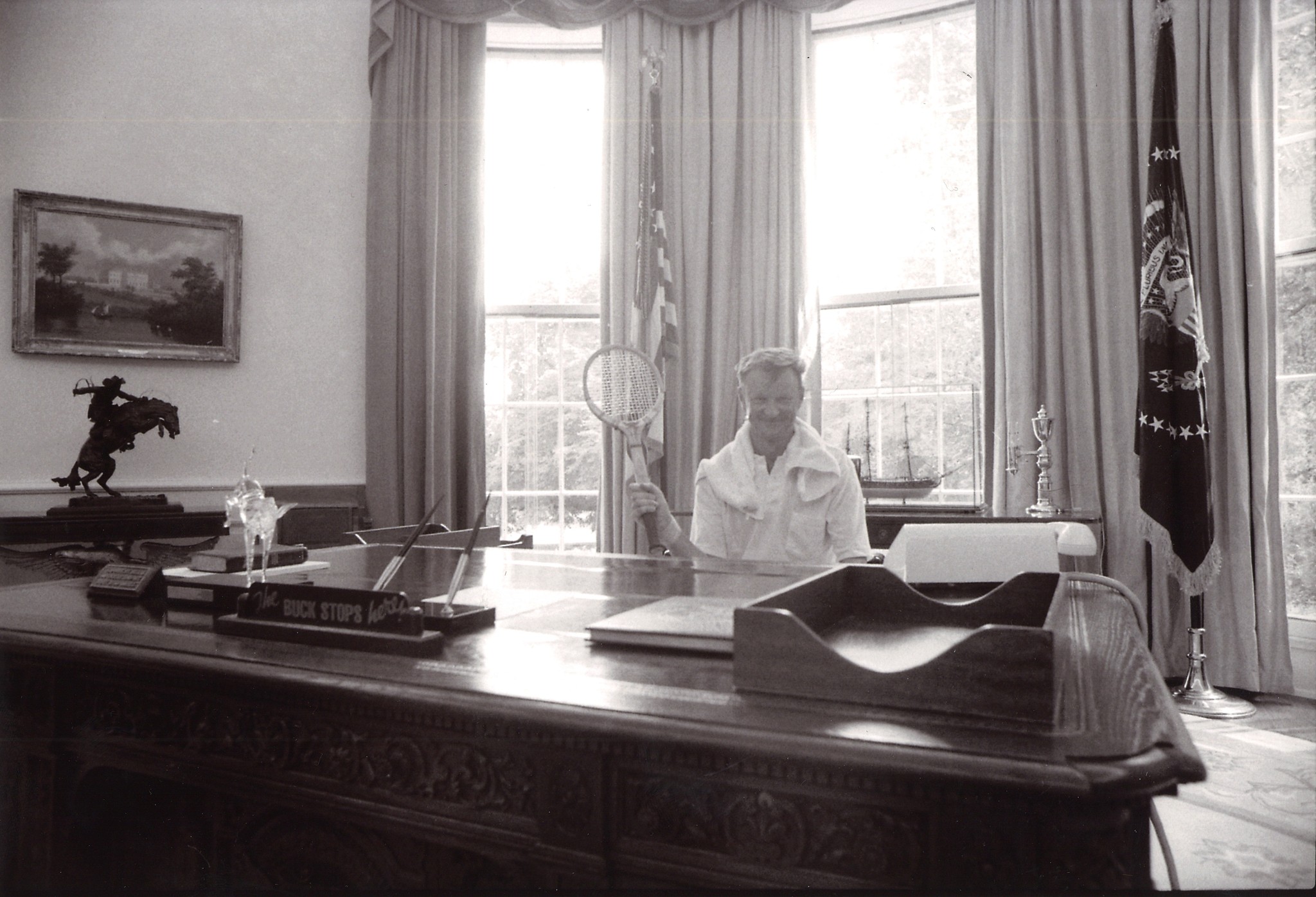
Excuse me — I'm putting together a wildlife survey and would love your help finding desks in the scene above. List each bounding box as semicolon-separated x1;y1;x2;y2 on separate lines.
864;508;1102;525
0;544;1207;893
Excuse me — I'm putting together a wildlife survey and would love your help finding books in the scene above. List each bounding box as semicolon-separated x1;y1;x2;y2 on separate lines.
187;544;308;572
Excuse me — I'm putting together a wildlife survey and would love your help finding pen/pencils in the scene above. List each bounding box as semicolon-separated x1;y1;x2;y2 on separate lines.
372;495;444;592
439;493;489;619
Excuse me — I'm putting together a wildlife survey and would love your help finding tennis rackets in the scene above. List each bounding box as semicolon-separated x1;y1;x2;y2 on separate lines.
582;342;673;556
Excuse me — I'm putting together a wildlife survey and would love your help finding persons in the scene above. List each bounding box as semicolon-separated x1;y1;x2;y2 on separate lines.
629;347;872;566
73;372;147;451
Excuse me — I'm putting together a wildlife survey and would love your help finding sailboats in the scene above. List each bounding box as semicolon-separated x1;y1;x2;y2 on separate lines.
839;397;974;502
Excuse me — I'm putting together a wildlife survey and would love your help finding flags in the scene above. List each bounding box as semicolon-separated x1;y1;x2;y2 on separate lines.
1132;1;1221;597
626;73;682;550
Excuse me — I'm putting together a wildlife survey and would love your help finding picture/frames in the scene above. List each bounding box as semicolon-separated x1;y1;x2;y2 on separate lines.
13;188;244;364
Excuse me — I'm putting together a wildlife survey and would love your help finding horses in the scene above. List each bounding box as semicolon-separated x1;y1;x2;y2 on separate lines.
51;395;181;497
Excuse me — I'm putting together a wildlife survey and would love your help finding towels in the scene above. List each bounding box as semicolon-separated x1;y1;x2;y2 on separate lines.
694;415;842;520
